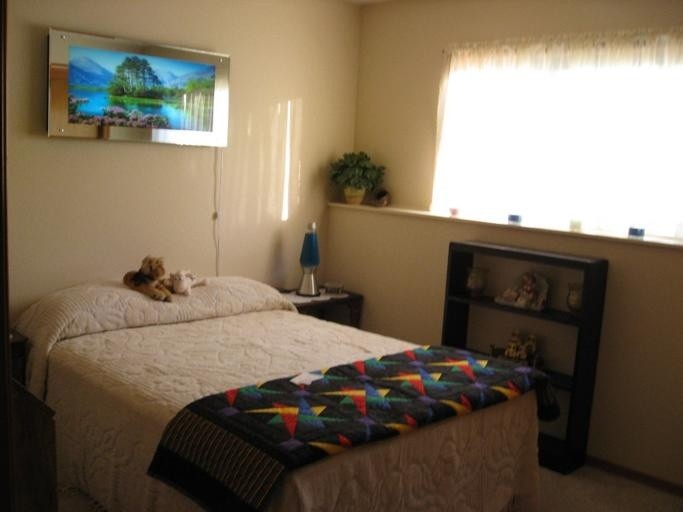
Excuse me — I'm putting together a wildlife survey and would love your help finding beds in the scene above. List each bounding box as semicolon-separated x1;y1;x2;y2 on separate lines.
21;276;538;508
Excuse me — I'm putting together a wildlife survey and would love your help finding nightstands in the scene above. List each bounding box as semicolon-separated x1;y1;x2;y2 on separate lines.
279;285;364;325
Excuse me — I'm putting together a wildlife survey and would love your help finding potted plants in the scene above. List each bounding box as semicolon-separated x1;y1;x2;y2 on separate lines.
328;147;386;207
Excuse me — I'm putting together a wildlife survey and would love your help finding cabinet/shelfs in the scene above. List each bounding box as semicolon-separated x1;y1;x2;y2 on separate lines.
439;240;610;474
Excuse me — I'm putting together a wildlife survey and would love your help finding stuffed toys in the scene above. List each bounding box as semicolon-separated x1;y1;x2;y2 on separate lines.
162;271;209;296
122;256;174;302
